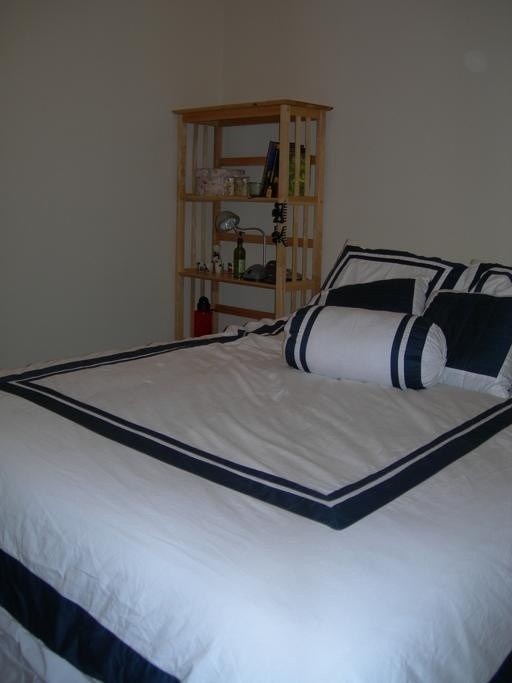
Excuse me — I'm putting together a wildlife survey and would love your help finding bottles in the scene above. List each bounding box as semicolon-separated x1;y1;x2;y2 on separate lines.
224;176;249;196
232;237;246;277
193;296;213;337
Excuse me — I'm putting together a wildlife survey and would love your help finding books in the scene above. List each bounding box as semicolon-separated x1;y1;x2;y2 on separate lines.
262;140;309;198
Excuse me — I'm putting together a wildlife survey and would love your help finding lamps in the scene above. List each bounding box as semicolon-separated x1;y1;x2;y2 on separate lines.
213;210;268;268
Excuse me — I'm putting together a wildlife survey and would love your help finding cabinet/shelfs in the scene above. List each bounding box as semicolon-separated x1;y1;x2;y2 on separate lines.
171;98;334;342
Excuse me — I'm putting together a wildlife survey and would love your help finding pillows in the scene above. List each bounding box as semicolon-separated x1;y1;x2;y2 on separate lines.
281;239;511;403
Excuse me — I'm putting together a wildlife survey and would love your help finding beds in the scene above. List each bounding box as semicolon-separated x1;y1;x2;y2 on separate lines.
0;310;512;683
